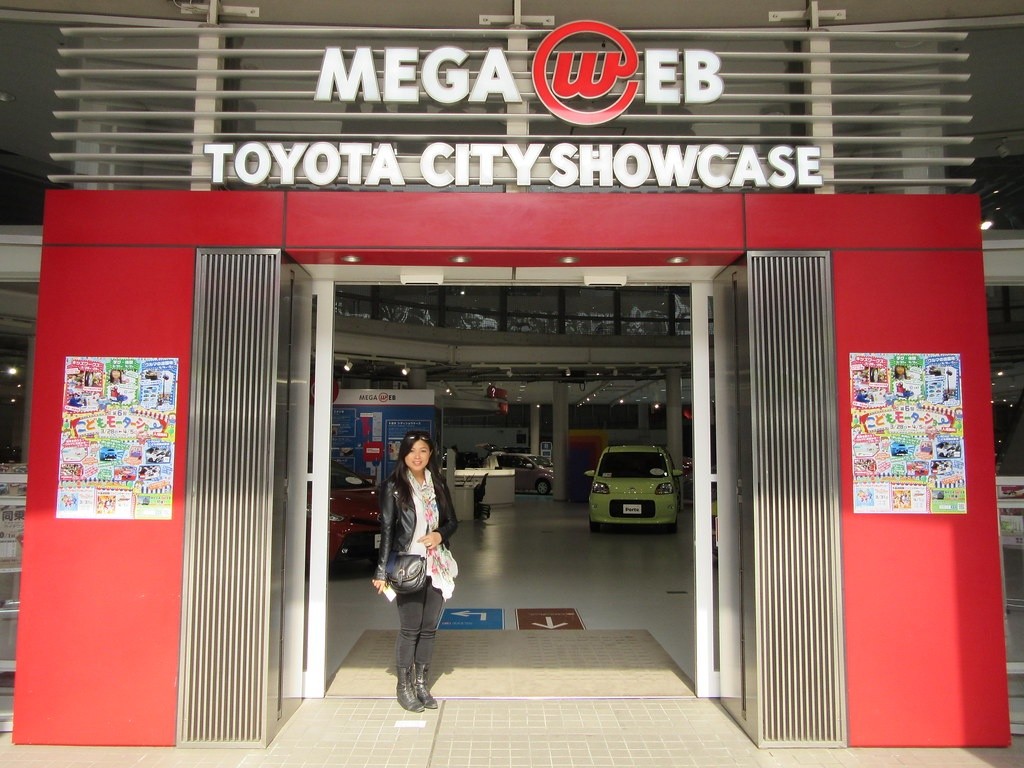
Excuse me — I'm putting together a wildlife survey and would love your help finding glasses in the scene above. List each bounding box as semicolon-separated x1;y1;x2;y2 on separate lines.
406;434;431;441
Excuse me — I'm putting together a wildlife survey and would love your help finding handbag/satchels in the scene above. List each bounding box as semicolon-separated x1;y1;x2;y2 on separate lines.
385;549;427;594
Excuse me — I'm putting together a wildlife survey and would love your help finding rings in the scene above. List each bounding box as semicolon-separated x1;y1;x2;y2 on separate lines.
429;543;431;545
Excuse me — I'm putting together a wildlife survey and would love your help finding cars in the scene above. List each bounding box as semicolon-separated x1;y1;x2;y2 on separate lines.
305;452;394;577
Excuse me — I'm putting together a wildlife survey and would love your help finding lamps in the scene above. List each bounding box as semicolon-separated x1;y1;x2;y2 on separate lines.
344;359;353;371
395;361;411;375
499;367;513;378
558;367;570;376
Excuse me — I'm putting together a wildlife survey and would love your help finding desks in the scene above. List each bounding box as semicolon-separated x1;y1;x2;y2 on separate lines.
454;482;479;520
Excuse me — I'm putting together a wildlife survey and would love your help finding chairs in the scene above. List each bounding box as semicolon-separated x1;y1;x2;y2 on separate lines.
474;472;492;517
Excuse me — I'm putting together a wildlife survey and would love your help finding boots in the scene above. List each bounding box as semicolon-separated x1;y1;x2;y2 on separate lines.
396;664;425;713
413;660;438;709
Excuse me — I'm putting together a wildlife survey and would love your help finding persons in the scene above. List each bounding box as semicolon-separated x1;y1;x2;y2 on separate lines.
372;430;457;712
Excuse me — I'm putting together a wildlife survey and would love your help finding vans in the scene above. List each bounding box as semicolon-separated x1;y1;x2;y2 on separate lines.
474;442;552;496
583;443;683;534
